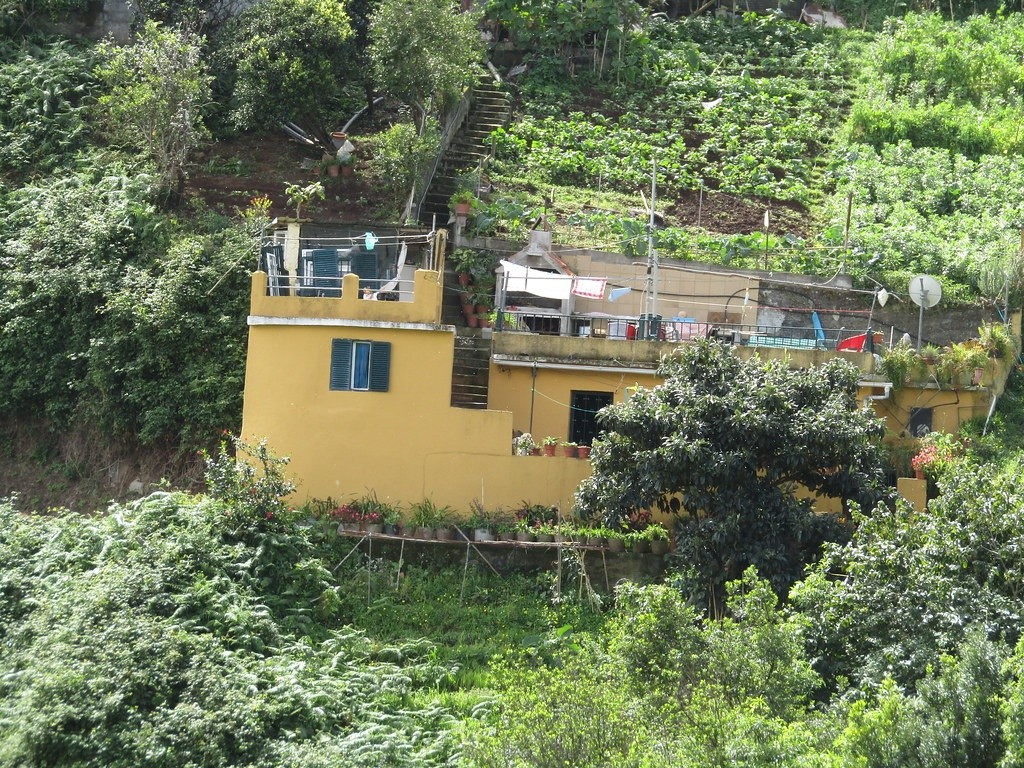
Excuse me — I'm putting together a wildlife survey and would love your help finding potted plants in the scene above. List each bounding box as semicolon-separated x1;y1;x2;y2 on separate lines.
450;250;521;329
312;156;356;177
519;440;530;455
907;339;988;385
451;181;471;216
560;442;576;457
532;444;541;454
873;327;885;343
542;435;560;456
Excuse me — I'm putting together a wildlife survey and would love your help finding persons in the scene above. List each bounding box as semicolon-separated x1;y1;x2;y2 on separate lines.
363;285;373;299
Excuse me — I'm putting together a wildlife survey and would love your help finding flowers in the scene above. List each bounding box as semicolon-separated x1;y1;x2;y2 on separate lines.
298;491;668;537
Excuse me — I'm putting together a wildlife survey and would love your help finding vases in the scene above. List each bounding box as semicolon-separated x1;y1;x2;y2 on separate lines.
578;446;592;458
304;521;667;556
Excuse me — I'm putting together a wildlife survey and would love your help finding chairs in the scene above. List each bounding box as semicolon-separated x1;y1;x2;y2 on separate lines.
837;333;866;352
